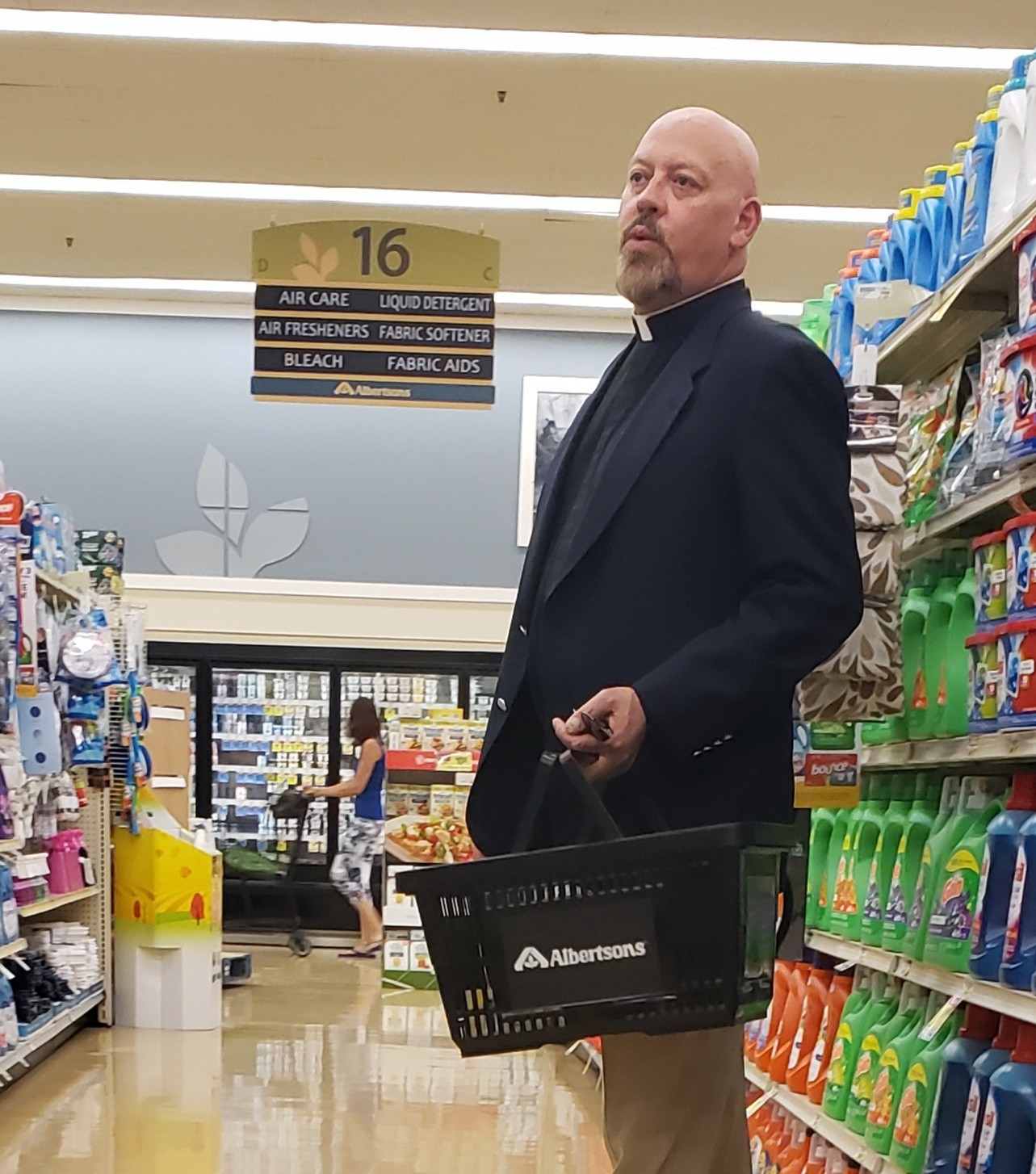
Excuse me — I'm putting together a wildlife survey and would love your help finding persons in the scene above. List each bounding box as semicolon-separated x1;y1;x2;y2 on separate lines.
466;102;866;1174
303;698;386;958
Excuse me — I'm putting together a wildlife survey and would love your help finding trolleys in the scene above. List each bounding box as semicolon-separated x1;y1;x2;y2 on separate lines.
216;789;314;957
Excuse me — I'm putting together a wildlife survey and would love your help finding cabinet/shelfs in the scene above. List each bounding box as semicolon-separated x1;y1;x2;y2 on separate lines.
146;659;499;925
0;555;129;1091
566;195;1036;1174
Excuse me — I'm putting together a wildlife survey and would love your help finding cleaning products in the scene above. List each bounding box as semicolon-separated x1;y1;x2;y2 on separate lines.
741;50;1036;1174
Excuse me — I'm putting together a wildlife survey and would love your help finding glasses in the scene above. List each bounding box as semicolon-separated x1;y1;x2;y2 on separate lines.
570;709;614;765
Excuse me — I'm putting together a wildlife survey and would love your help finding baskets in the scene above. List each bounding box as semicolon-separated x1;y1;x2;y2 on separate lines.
394;752;798;1059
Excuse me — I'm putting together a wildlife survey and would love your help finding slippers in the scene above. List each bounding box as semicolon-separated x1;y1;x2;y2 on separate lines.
338;945;382;958
